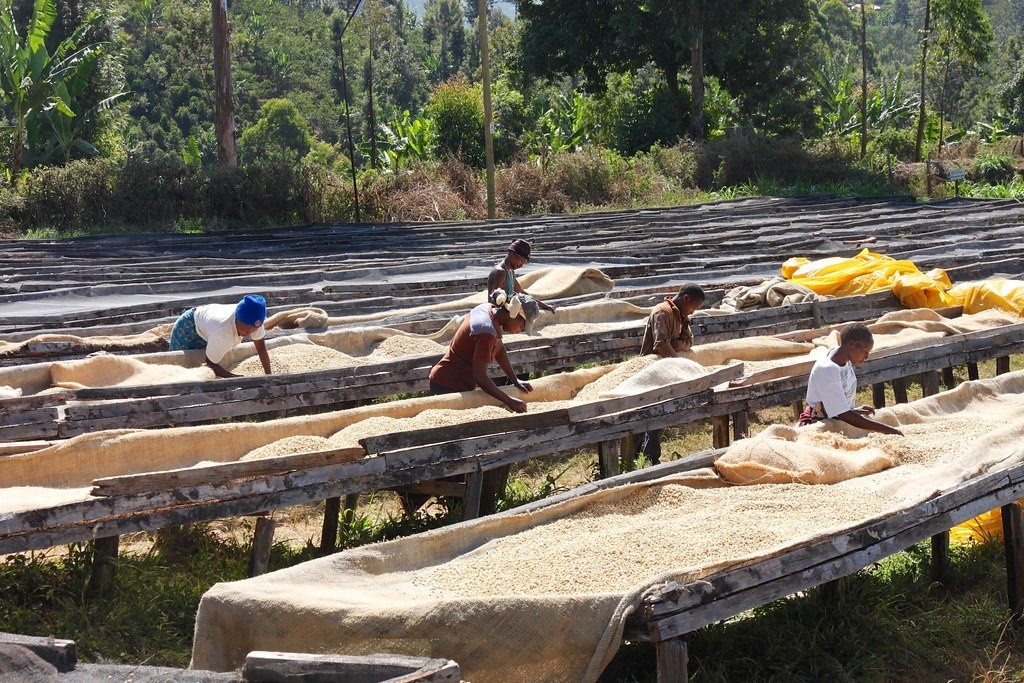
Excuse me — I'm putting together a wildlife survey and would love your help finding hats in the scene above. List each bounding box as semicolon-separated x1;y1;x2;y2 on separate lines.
508;238;531;262
519;293;541;325
235;294;267;328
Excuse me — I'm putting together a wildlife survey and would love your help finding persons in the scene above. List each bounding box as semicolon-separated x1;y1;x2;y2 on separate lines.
798;322;905;438
632;282;706;465
169;294;272;378
428;289;541;414
488;238;556;315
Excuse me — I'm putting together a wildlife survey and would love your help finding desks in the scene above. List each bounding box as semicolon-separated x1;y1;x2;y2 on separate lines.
0;196;1024;683
